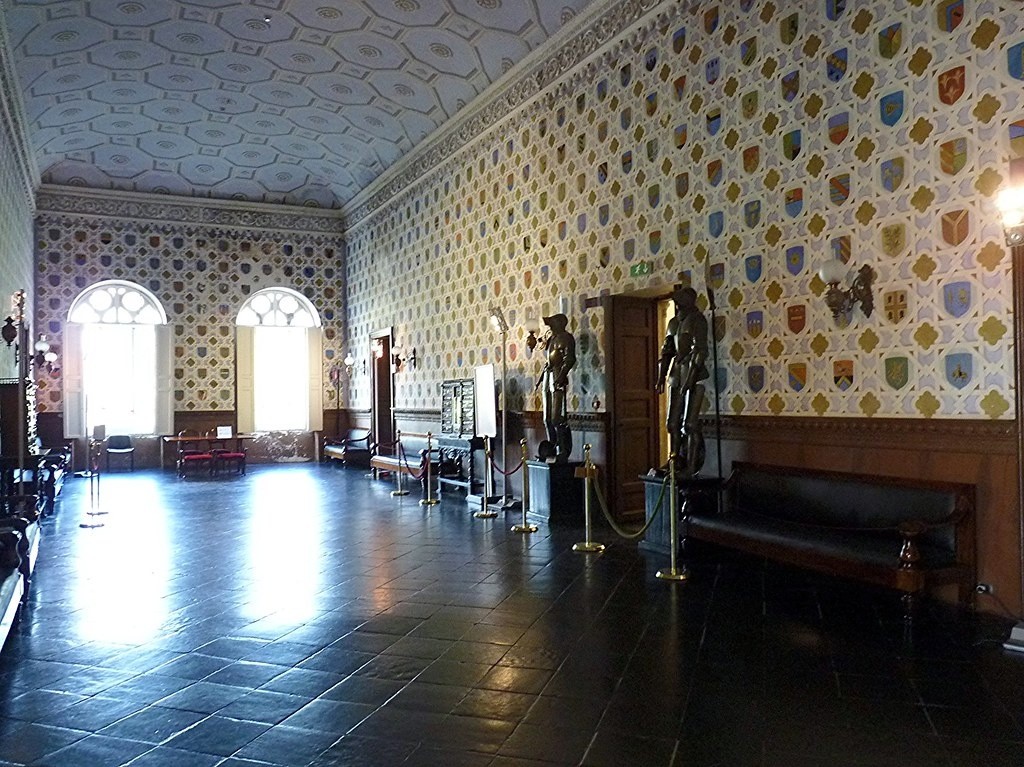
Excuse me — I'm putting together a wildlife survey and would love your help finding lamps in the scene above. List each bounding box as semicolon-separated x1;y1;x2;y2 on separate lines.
524;319;553;353
486;306;520;512
991;181;1024;657
819;258;874;321
15;333;50;369
1;316;18;347
30;352;57;374
391;345;417;370
344;352;366;379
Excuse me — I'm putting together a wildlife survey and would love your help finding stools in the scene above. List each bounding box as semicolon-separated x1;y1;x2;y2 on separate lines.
181;453;213;479
215;453;247;477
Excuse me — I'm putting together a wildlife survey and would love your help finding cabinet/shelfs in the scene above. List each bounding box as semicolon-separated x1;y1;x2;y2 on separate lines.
0;378;39;457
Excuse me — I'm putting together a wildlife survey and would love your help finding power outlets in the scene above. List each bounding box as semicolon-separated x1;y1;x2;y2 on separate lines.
976;584;993;595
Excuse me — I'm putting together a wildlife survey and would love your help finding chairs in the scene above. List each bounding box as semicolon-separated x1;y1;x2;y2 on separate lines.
106;435;135;473
0;495;44;585
9;445;71;516
205;428;233;477
177;428;208;480
0;527;26;655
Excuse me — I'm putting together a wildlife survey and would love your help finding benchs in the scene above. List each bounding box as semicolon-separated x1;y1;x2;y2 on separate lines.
370;429;457;488
679;461;977;664
324;427;372;470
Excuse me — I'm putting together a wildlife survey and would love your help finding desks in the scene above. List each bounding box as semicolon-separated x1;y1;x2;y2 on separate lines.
163;435;257;478
434;434;496;494
0;449;53;529
